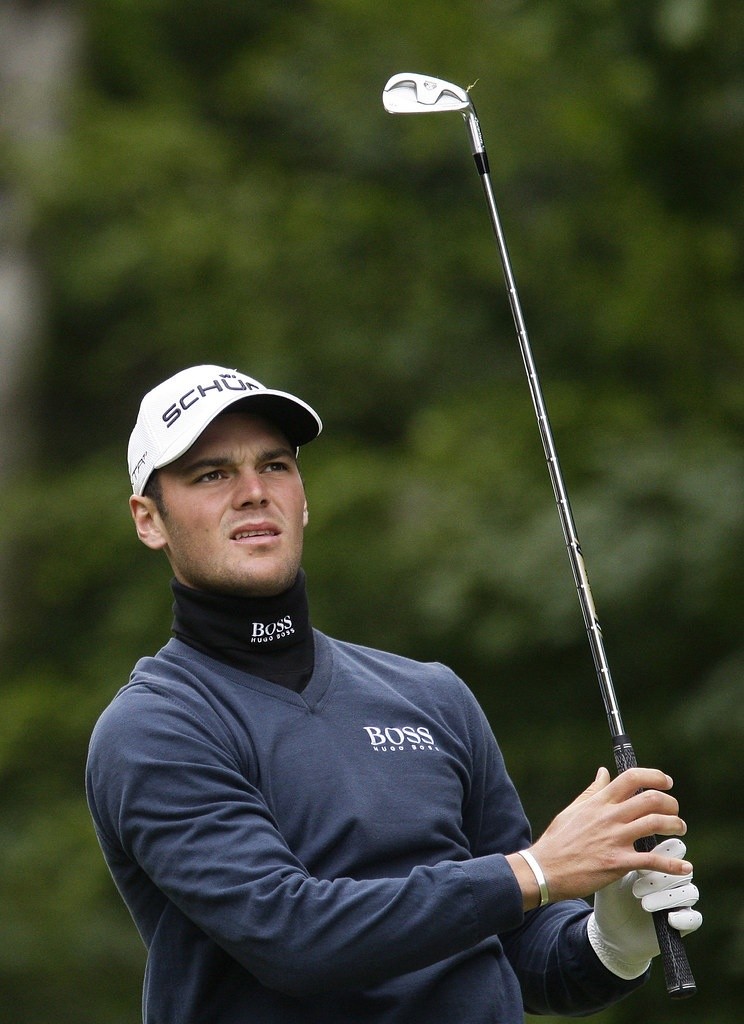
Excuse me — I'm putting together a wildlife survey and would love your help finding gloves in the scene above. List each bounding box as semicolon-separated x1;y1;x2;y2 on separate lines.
588;839;703;981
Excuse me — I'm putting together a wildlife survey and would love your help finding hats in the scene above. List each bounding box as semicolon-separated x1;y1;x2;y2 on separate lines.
128;364;322;494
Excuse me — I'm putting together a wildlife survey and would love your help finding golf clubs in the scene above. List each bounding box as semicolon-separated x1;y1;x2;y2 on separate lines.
380;68;703;1002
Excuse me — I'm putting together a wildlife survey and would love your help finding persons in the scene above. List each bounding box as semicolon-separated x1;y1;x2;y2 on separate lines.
85;362;704;1024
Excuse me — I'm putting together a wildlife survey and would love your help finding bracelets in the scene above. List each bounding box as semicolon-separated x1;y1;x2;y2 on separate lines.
517;849;549;909
